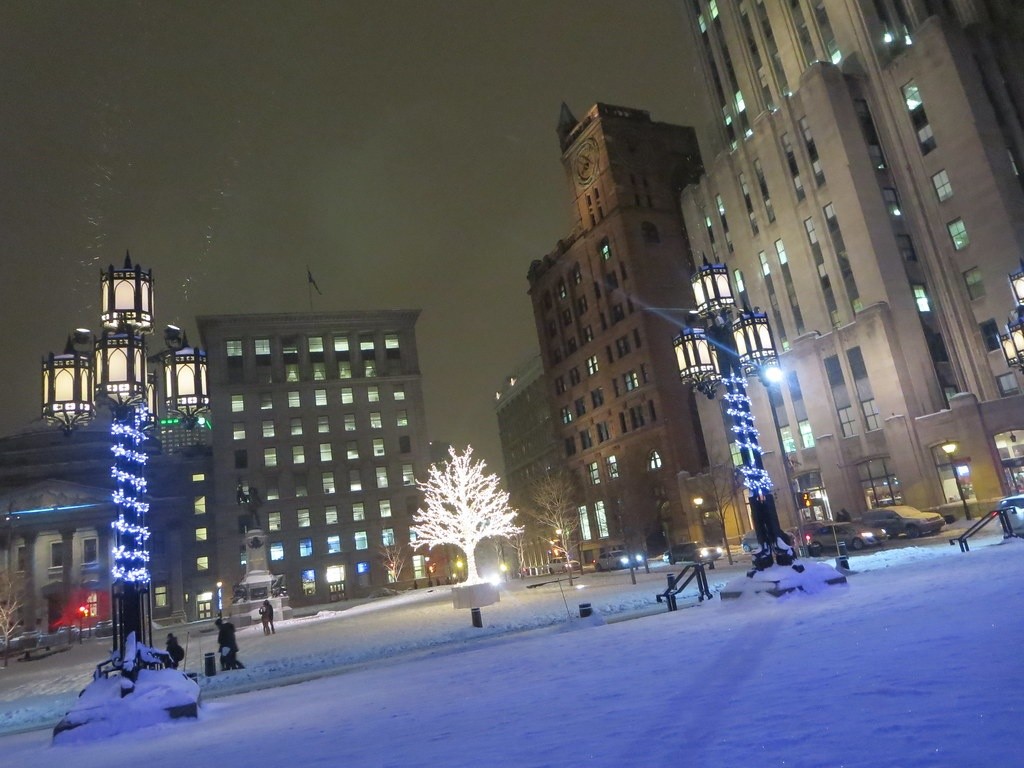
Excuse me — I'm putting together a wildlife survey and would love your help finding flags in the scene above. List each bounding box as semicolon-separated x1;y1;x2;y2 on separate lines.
308;269;322;296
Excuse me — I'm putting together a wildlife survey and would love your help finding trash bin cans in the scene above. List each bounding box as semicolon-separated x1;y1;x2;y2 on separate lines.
579;603;593;618
204;652;217;676
944;514;955;524
838;541;849;559
810;542;821;558
835;556;850;569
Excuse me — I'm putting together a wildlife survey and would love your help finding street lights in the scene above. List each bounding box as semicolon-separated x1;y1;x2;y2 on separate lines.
693;497;715;570
941;442;972;521
425;555;433;587
215;582;222;619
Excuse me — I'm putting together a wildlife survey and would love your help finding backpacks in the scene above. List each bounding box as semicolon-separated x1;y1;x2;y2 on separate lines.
173;646;184;660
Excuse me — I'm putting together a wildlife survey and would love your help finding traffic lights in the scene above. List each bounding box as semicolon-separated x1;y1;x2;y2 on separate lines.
798;490;812;509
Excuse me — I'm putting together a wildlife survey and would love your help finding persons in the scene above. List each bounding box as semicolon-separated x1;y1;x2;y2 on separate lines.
259;600;276;636
836;512;845;522
244;487;263;528
215;619;239;671
841;508;852;522
166;633;179;670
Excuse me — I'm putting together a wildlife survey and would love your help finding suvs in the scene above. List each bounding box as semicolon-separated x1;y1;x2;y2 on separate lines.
549;557;581;575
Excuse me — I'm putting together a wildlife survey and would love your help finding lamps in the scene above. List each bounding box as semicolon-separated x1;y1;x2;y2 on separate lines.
164;324;182;341
70;326;94;345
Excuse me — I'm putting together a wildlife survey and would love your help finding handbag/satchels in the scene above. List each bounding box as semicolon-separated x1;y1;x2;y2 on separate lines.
221;646;230;656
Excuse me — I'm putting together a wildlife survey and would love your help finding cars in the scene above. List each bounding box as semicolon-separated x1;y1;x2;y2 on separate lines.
57;625;81;635
860;505;946;539
789;520;888;556
21;629;42;640
741;528;796;553
96;620;113;630
999;494;1024;537
592;549;645;572
663;541;723;566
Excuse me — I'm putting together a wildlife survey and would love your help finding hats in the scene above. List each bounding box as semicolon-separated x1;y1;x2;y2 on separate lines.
215;619;222;625
